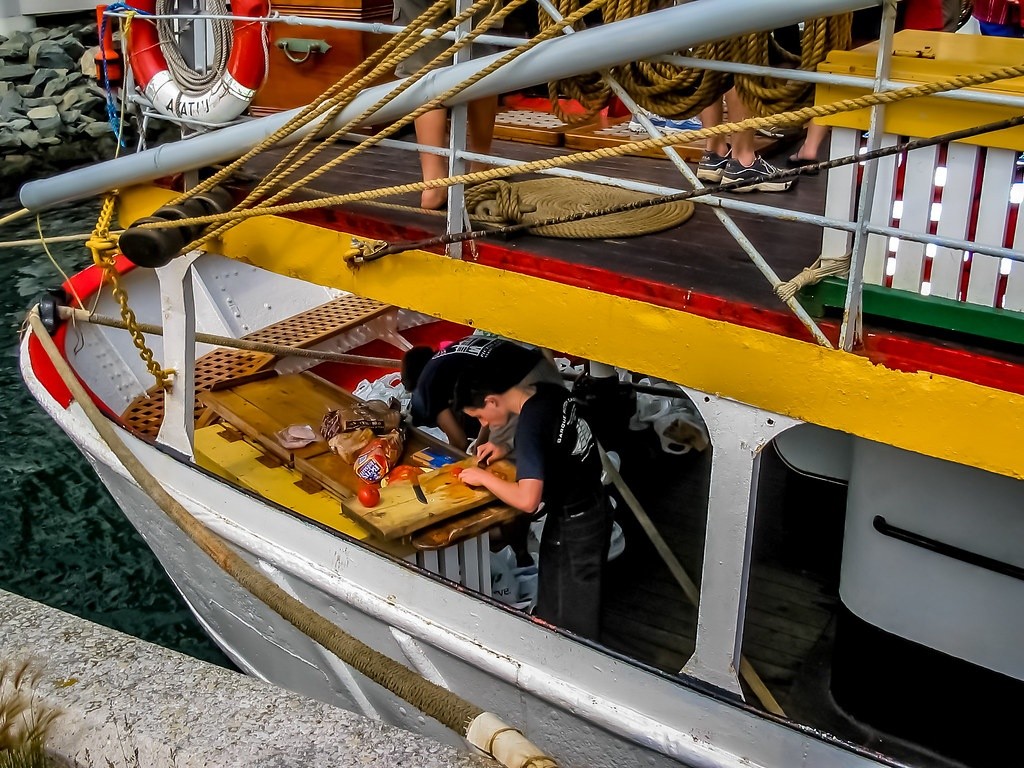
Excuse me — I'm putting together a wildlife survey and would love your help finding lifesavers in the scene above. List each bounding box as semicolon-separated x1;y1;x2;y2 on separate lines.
120;2;276;122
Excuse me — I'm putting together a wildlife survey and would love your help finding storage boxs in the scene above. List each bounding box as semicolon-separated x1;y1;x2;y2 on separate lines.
251;0;395;118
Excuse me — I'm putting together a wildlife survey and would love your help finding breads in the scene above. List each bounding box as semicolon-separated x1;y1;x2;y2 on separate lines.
337;433;399;484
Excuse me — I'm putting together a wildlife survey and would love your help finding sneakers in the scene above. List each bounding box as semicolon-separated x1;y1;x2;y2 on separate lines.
697;149;801;192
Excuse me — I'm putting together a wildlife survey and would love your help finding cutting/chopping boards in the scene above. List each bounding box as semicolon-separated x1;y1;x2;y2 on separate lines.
341;455;517;541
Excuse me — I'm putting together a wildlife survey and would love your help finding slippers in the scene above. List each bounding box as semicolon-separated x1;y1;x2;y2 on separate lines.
786;150;819;176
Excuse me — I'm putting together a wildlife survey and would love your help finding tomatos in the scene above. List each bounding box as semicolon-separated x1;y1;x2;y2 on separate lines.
358;485;380;507
385;465;424;481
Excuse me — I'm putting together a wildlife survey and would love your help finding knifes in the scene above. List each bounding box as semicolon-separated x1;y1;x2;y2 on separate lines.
409;471;427;504
468;454;492;490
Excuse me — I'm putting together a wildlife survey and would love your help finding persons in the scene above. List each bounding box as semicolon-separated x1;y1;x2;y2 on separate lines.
787;0;945;175
402;334;616;643
629;83;799;193
392;1;503;210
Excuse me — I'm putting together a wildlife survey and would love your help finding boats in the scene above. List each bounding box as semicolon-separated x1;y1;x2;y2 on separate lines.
19;1;1024;768
